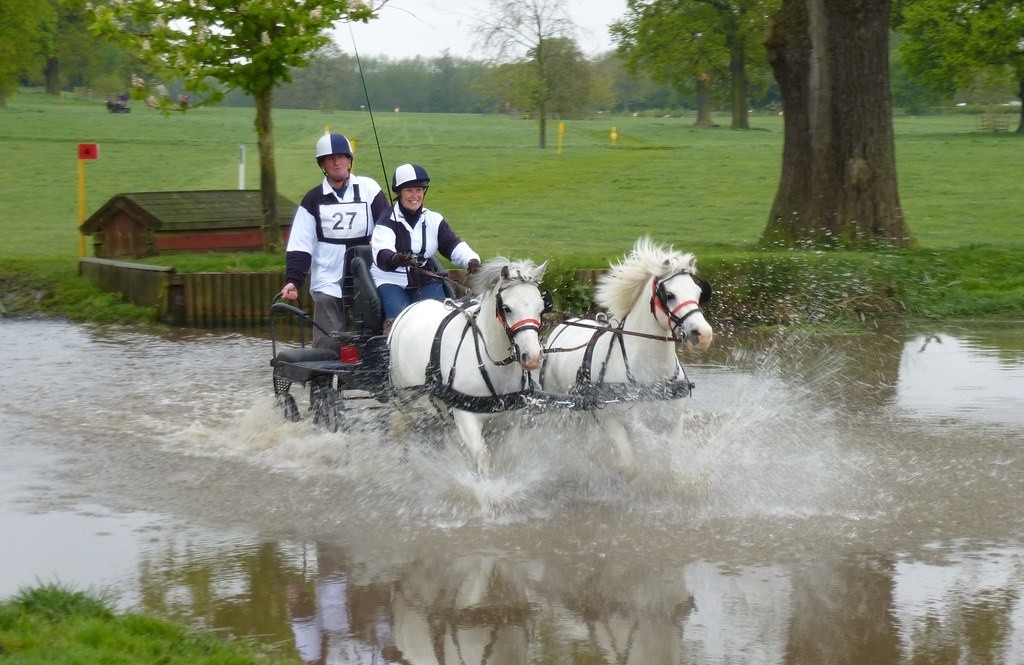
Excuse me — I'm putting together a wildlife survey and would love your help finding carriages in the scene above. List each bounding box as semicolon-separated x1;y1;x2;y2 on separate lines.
267;234;716;476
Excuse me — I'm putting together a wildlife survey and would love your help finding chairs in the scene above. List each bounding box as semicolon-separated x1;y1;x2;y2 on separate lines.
348;245;454;331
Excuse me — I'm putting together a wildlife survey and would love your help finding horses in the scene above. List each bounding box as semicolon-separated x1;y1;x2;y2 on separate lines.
539;231;714;471
387;255;549;478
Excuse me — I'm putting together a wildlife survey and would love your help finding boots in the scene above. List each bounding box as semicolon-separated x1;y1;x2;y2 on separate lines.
383;318;395;335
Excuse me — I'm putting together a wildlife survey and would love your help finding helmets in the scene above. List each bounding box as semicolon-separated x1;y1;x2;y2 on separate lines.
316;133;353;157
392;163;430;191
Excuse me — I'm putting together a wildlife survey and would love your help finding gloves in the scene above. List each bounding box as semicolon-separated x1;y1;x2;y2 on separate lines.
468;259;482;273
395;254;416;267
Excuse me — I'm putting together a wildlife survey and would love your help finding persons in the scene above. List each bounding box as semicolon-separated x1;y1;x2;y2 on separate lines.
280;132;390;422
369;163;483;337
